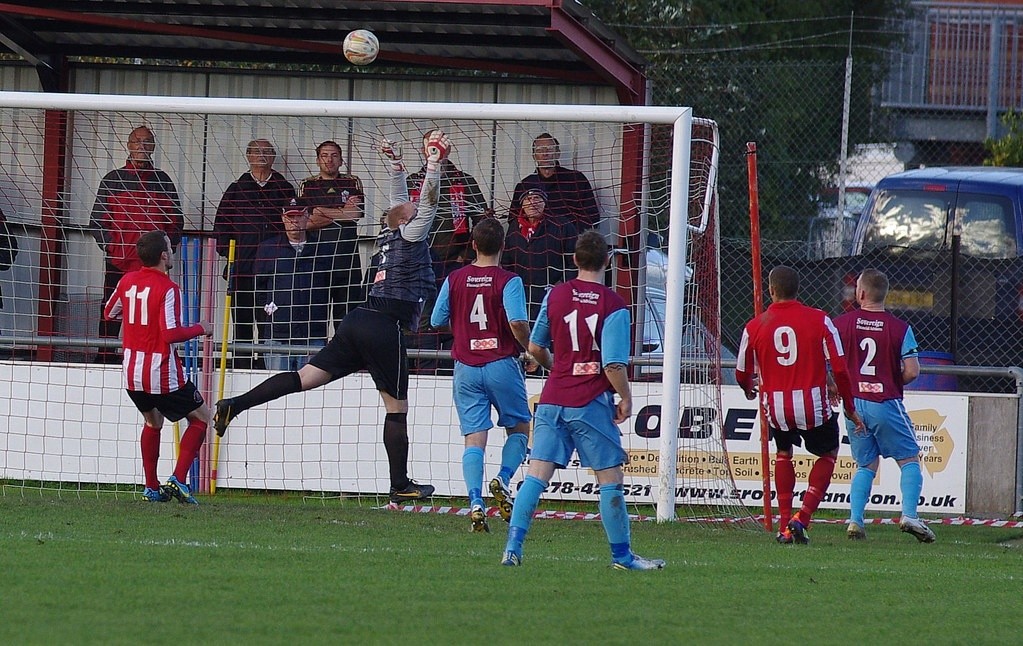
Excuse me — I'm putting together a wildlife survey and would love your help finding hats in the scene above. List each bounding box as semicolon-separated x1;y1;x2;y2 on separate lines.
281;196;308;215
517;175;549;205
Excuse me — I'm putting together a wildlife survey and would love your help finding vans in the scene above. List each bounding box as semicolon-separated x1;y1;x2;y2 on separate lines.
804;181;874;307
640;232;739;386
843;168;1023;392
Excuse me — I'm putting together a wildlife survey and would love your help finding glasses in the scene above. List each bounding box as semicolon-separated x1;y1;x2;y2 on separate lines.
521;199;544;204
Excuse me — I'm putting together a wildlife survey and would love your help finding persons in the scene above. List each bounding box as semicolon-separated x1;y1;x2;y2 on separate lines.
213;138;365;372
406;130;601;334
502;232;665;571
829;267;936;544
88;126;184;352
103;231;213;504
431;218;537;532
735;265;866;543
213;131;450;504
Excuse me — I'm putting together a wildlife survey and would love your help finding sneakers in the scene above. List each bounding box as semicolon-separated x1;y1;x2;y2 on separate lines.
163;475;198;504
489;477;514;523
788;516;809;544
847;523;868;541
213;398;239;438
501;551;522;566
899;514;935;544
776;529;794;544
469;504;493;533
390;478;435;505
142;483;164;502
613;556;665;570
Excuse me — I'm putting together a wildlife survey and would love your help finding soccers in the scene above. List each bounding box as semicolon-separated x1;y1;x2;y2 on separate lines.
340;28;380;66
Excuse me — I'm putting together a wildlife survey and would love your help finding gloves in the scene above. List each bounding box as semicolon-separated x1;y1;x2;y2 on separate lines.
380;138;403;164
424;130;450;163
